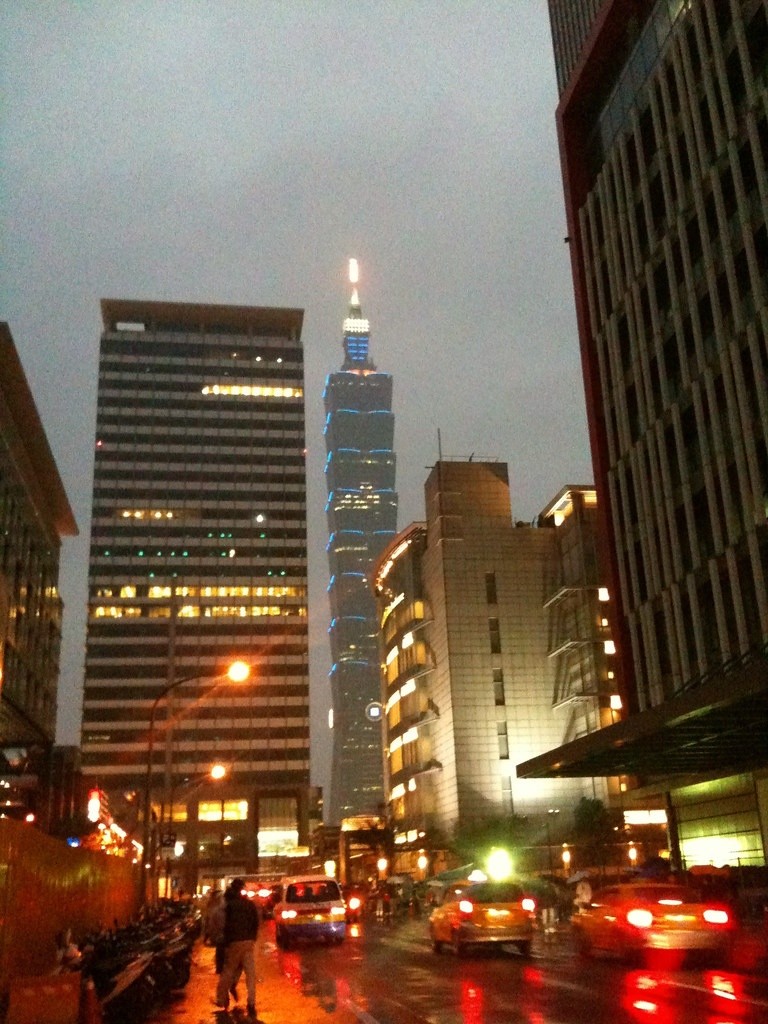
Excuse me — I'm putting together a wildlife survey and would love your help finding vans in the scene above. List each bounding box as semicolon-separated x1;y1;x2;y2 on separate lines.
273;874;348;949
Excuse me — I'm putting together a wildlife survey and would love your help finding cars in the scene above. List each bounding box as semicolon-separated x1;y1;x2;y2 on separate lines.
569;882;738;968
427;879;535;959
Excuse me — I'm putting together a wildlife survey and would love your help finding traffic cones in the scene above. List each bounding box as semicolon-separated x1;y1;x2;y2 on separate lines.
79;975;104;1024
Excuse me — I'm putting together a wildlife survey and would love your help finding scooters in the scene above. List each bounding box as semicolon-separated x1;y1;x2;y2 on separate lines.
58;896;203;1024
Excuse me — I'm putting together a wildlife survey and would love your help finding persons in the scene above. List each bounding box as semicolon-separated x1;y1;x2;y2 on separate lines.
202;879;259;1016
530;878;592;935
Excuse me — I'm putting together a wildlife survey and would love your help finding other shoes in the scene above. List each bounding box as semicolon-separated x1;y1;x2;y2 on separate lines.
245;1005;256;1019
230;989;238;1004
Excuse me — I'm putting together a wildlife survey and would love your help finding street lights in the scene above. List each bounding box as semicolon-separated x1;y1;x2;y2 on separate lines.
137;658;250;908
163;765;226;900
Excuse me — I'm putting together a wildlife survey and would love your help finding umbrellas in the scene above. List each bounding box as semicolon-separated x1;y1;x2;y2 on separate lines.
566;871;591;884
